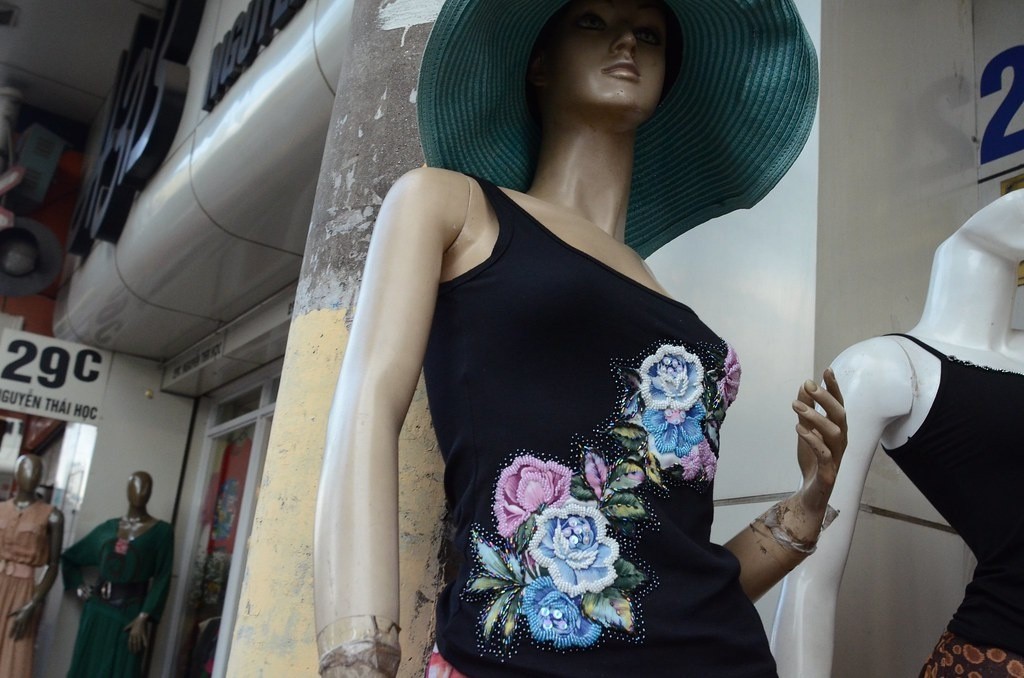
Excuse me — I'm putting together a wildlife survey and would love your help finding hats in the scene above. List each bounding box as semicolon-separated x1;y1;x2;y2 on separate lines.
417;0;822;256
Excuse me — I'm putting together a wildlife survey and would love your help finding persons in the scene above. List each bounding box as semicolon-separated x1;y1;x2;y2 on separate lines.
313;0;850;678
769;187;1023;678
1;454;65;678
62;471;175;678
